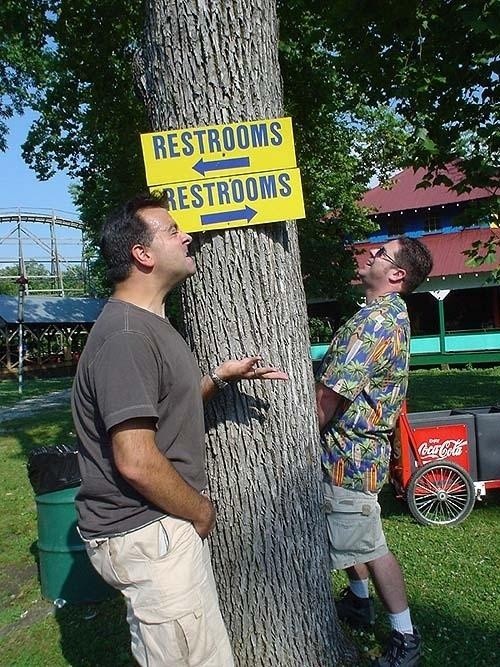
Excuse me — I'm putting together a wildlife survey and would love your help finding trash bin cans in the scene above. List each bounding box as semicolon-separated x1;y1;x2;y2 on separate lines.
28;443;112;604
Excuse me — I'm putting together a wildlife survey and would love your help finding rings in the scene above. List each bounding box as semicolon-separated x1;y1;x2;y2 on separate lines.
252;368;260;377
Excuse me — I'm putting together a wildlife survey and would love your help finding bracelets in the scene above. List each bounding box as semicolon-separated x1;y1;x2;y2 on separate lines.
209;372;229;393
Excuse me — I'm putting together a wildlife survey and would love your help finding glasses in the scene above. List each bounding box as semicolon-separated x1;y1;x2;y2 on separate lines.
373;245;409;275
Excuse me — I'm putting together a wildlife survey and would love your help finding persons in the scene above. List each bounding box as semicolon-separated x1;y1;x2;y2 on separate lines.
314;236;435;667
70;194;290;667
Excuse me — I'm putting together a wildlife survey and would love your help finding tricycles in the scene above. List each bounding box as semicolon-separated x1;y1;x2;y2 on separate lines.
389;399;500;527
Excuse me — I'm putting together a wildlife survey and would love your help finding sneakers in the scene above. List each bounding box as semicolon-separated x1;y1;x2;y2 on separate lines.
370;625;421;667
334;586;376;630
